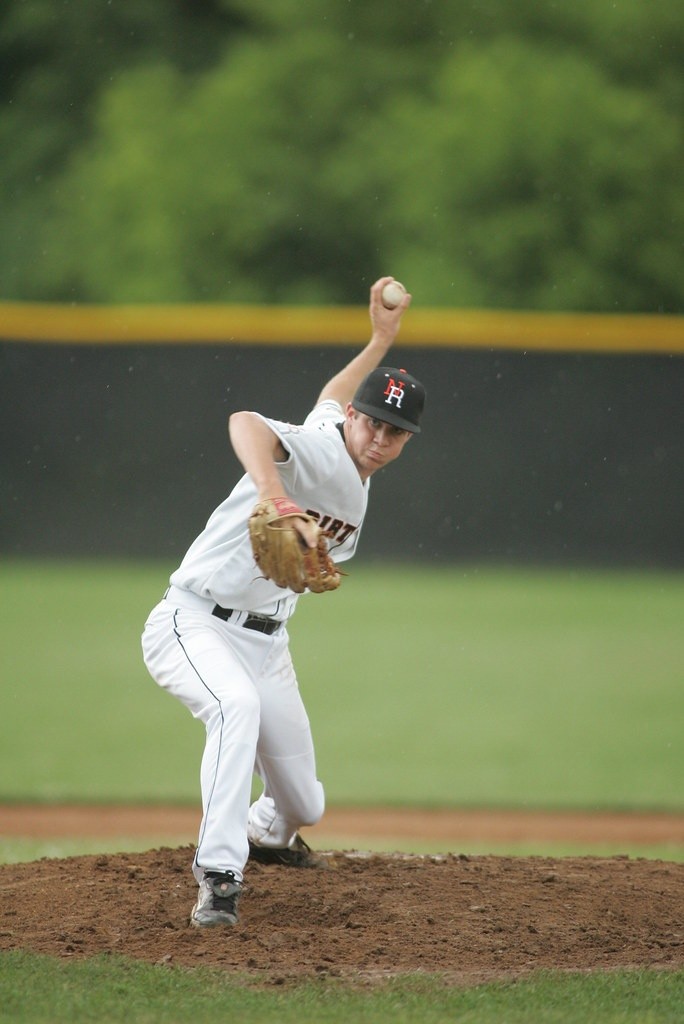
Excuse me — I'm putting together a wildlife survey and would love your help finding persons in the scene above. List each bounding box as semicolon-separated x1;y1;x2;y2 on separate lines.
138;277;424;927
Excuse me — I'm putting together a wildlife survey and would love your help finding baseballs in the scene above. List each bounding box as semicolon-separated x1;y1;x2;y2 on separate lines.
381;280;407;311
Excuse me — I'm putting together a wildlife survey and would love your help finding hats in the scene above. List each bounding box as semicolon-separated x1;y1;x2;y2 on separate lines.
351;367;425;433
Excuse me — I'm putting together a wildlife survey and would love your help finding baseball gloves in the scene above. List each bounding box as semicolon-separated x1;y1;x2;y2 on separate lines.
246;495;351;594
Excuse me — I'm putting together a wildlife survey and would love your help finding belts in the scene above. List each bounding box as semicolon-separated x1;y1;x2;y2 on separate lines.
163;585;281;635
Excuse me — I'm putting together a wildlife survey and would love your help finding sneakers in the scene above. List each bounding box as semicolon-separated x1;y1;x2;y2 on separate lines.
248;832;337;870
189;869;250;930
